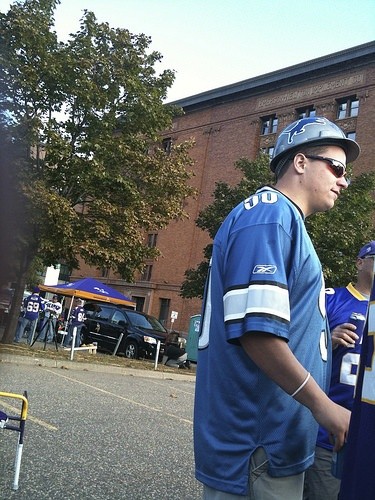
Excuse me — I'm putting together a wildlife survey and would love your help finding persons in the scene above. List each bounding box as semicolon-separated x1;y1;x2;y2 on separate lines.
194;117;360;500
15;286;85;349
155;343;192;370
321;244;375;500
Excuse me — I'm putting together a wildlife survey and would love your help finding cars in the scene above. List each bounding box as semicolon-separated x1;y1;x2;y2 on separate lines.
0;287;31;316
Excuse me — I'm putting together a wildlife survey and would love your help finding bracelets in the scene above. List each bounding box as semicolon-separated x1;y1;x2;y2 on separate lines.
291;371;310;397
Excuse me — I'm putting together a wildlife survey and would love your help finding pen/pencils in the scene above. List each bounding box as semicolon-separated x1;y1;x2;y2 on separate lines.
332;436;337;477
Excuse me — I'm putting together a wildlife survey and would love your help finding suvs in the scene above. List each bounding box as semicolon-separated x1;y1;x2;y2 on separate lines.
78;302;169;361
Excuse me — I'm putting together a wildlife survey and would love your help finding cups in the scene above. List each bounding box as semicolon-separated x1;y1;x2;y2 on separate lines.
346;315;365;347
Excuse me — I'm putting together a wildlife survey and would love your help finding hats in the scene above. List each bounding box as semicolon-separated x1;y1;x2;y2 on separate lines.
33;286;40;293
53;295;58;300
357;241;375;258
75;300;82;305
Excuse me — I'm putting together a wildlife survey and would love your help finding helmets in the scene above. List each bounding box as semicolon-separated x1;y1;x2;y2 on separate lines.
270;116;361;174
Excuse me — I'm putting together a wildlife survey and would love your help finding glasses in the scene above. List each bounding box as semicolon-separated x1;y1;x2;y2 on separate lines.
289;156;347;179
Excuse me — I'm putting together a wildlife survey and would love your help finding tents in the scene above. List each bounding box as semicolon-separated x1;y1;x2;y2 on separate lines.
29;277;136;347
